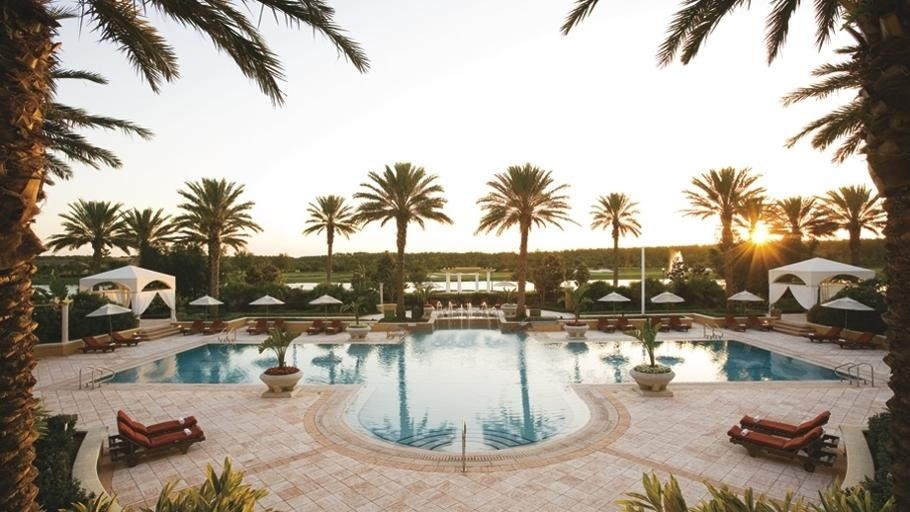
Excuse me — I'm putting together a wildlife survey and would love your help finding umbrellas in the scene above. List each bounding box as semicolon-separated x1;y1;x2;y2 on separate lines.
188;294;225;306
820;295;876;312
596;290;631;302
85;302;133;318
308;293;344;305
727;288;765;302
248;294;286;305
650;290;686;304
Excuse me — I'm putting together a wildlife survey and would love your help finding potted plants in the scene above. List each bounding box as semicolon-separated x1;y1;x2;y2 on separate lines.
416;285;436;320
623;317;675;397
258;326;303;398
552;283;596;338
500;288;518;321
340;296;372;339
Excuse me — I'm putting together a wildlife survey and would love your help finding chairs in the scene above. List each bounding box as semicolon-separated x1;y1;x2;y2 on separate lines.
808;325;845;343
108;422;206;467
179;320;225;336
107;330;142;346
727;425;838;472
79;336;121;352
108;410;198;446
726;317;747;331
246;318;286;336
838;332;880;350
750;314;775;331
306;319;346;335
597;314;691;332
739;410;840;451
